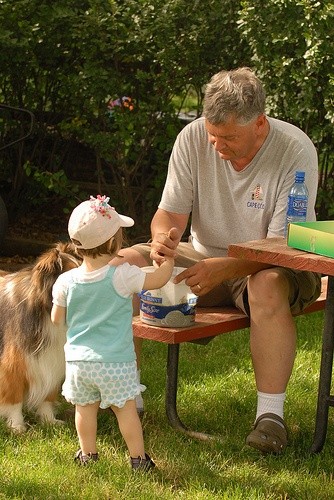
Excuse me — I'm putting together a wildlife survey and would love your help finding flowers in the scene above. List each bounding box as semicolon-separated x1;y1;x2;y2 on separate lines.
88;195;113;220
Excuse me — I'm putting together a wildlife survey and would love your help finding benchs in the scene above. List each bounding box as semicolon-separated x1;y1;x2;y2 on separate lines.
131;274;328;344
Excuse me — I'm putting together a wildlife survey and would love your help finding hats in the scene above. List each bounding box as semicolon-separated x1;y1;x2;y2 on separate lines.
67;195;134;250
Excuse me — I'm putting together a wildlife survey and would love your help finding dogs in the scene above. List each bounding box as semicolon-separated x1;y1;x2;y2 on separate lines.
0;241;84;435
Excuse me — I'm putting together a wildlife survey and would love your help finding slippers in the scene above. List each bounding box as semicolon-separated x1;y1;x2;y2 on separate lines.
245;411;291;456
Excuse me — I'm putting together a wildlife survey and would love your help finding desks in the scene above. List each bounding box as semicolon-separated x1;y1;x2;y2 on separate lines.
164;239;334;456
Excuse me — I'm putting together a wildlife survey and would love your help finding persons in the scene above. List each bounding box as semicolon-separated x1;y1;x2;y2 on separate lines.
51;194;174;474
64;68;322;455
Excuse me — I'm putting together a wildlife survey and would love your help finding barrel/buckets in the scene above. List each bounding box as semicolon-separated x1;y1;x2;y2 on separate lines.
139;266;198;328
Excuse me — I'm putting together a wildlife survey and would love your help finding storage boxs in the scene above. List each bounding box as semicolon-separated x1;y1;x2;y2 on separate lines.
287;220;334;259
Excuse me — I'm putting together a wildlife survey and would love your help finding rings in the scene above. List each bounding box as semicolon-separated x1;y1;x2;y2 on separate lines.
197;285;200;288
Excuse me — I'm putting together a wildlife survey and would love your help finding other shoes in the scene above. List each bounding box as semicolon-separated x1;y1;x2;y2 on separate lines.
130;453;160;478
73;449;98;468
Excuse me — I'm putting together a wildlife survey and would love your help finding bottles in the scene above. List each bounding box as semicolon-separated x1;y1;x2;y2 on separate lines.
285;170;310;238
